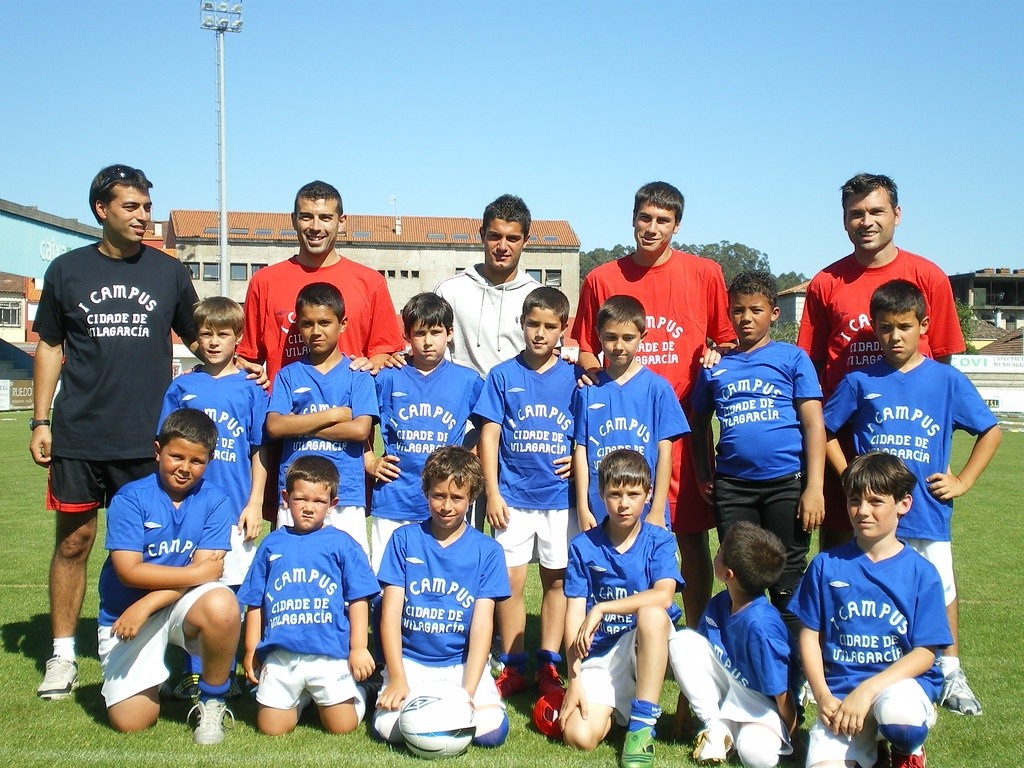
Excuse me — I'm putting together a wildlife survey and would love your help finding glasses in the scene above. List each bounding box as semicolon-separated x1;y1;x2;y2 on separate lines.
102;166;148;189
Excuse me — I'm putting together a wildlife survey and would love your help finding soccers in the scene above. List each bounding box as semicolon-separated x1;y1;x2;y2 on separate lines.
398;680;477;759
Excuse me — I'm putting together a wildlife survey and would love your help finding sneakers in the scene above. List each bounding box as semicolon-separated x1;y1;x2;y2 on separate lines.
890;742;924;768
693;726;735;762
620;726;657;768
36;654;80;703
854;739;891;768
495;666;530;698
223;671;242;698
490;635;503;662
937;668;984;717
358;661;386;718
535;663;567;695
165;670;202;699
186;698;236;745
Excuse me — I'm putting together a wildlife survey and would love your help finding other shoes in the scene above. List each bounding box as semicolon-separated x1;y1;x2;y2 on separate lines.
798;685;809;709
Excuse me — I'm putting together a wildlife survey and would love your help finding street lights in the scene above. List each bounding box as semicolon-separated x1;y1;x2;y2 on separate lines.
199;0;244;299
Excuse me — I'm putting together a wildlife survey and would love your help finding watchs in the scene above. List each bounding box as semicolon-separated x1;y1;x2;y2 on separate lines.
29;418;51;431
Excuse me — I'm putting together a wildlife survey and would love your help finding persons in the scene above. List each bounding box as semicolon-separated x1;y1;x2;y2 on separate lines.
97;409;241;744
265;280;380;564
570;180;740;630
570;294;691;532
822;278;1002;715
373;445;509;748
797;173;966;550
668;521;807;768
786;452;954;768
687;271;827;710
363;292;485;664
237;455;381;736
558;449;686;768
235;180;406;532
472;286;586;698
156;296;273;700
379;193;581;533
29;165;270;700
